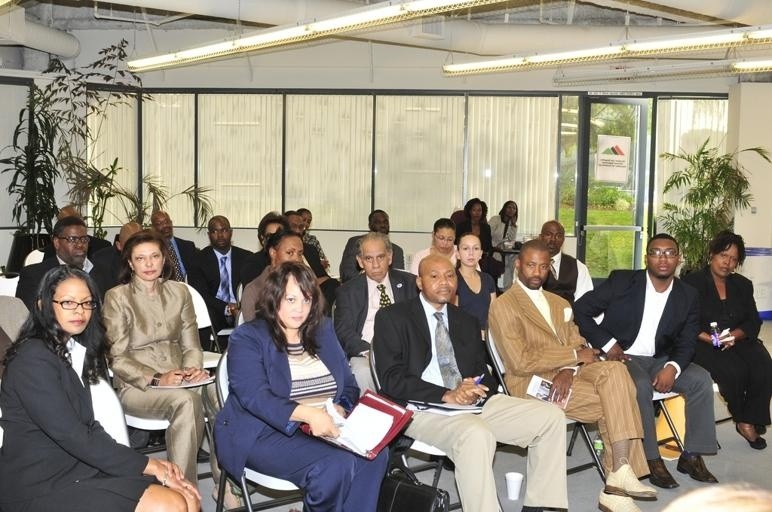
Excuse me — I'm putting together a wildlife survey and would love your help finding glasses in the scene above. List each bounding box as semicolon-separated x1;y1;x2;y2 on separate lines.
57;235;90;243
289;223;307;231
645;247;681;259
51;299;97;310
434;233;455;242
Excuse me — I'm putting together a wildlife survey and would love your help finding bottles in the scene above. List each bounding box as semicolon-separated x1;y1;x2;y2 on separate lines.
592;432;604;472
710;322;722;348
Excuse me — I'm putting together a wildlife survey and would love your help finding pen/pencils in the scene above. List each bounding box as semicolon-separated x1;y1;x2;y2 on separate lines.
588;342;603;362
474;373;485;385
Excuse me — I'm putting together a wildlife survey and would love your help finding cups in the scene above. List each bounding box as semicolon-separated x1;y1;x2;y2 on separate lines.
505;471;524;499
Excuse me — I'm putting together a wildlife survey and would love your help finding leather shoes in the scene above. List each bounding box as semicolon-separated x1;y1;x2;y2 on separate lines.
736;422;767;450
756;424;767;435
598;488;642;512
678;455;720;484
605;457;658;499
648;460;679;489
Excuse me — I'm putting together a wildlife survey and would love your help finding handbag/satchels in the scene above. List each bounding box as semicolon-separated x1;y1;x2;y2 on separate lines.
377;464;450;512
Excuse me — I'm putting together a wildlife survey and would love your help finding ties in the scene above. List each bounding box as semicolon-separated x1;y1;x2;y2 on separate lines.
549;259;558;280
219;256;230;302
434;310;463;391
166;239;184;283
377;284;391;309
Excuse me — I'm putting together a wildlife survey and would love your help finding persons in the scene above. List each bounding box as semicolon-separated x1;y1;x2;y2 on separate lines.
490;232;772;511
376;199;592;512
0;205;417;512
